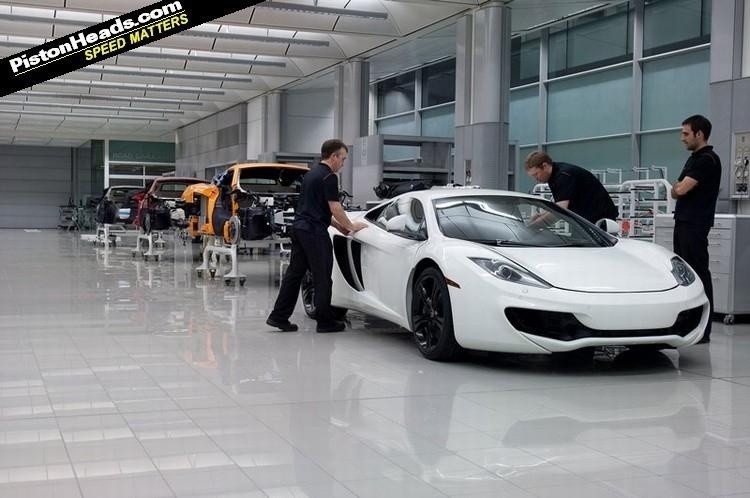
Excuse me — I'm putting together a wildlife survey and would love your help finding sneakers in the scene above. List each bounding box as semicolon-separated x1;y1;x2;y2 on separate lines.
696;336;710;344
316;319;346;332
266;314;297;331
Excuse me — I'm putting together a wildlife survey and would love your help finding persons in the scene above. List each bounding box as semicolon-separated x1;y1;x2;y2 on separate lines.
671;114;723;345
266;138;370;335
523;150;619;235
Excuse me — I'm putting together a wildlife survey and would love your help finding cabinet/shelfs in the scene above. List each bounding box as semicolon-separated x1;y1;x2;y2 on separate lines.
654;213;750;325
